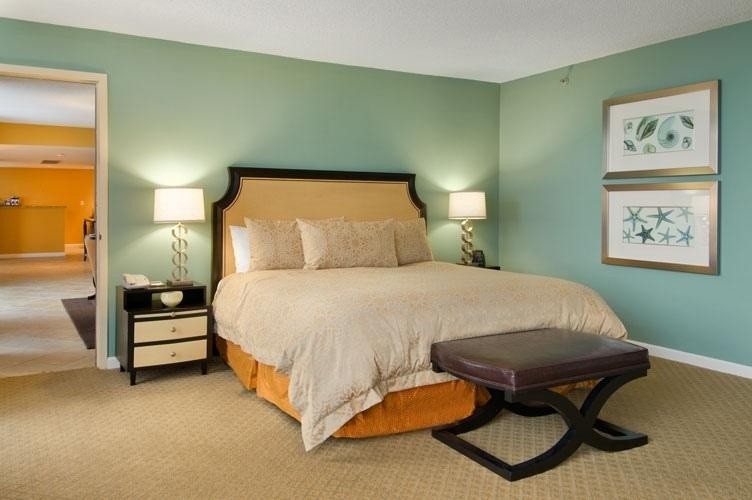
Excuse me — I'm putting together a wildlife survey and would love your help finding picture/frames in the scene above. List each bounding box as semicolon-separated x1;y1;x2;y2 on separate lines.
601;180;720;276
601;79;720;179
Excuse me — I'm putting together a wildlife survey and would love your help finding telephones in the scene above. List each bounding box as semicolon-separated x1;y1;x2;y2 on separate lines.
121;273;150;289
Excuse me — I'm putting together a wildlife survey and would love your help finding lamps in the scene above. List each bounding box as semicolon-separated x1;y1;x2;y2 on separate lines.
448;191;488;266
153;188;205;287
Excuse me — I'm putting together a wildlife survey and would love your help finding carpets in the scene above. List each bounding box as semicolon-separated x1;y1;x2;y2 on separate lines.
62;297;95;349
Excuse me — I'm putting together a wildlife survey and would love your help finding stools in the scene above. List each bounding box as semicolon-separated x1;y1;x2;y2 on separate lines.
431;327;653;482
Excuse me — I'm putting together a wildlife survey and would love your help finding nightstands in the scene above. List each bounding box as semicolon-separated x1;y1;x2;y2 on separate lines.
115;280;213;386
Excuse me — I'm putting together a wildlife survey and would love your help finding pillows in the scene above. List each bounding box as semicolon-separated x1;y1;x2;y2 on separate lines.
296;218;399;271
393;218;435;268
229;224;252;275
243;215;345;275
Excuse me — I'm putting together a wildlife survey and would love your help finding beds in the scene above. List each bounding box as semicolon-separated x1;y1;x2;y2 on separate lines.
211;166;602;440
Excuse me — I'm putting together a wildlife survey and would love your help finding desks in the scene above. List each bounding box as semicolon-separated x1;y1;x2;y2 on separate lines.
478;265;501;270
